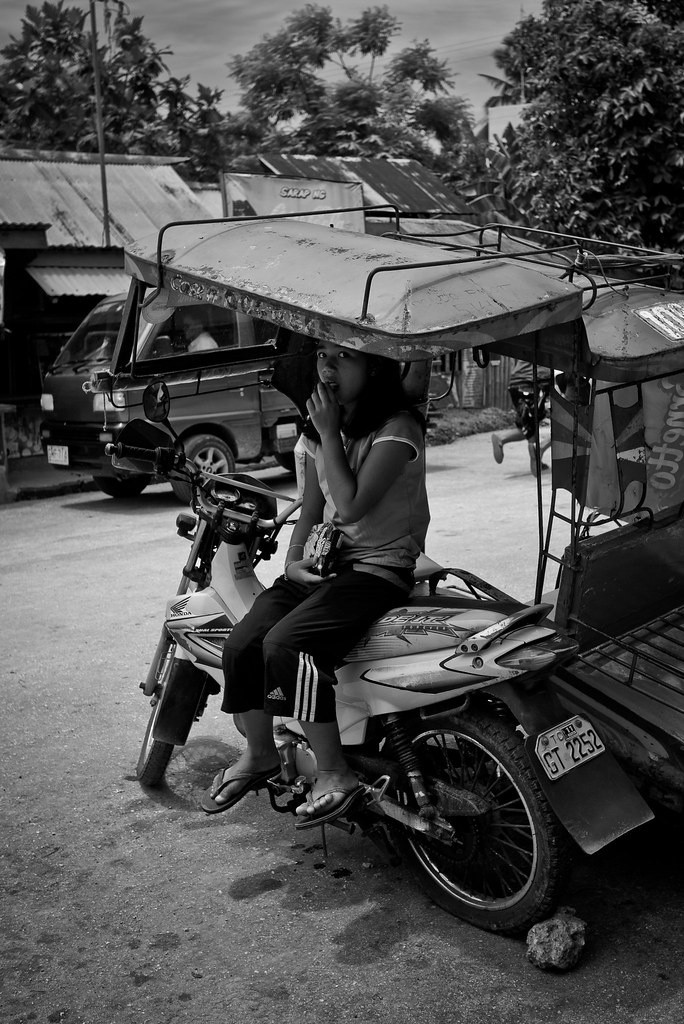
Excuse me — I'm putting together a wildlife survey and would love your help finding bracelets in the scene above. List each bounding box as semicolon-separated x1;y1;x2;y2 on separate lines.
284;561;297;582
286;544;304;554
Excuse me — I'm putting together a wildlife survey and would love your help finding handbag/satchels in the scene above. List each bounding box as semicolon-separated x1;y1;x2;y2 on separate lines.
302;522;344;578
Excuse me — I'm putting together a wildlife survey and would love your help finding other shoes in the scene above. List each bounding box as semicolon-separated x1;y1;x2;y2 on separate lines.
491;433;504;464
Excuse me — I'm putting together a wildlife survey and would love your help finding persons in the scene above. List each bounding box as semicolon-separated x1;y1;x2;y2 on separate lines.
175;307;220;359
200;339;431;830
492;357;552;478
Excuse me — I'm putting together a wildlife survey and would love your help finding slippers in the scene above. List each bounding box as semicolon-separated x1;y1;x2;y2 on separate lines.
201;762;282;814
294;784;365;831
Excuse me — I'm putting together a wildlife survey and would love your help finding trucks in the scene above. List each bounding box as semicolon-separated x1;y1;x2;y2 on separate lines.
36;286;308;505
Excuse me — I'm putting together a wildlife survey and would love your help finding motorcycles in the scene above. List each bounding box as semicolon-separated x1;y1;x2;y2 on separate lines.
100;373;656;932
